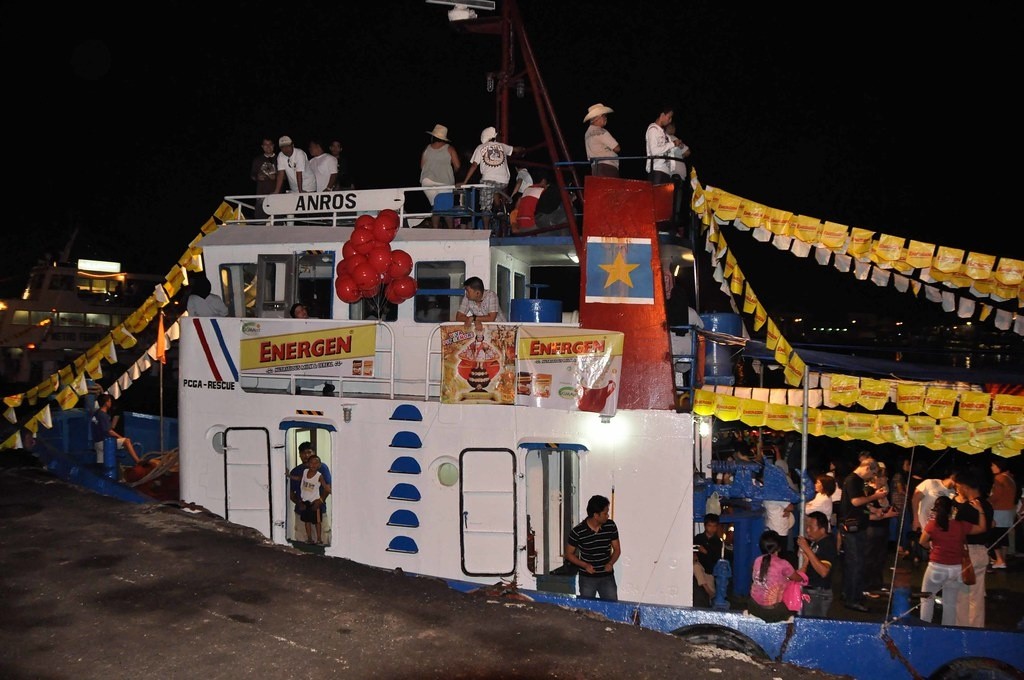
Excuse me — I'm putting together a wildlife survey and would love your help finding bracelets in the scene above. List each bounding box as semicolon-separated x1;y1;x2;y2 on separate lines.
319;498;323;503
473;315;476;321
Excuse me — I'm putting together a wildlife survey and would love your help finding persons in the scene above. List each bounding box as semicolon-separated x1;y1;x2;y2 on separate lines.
290;303;335;394
715;450;1017;628
110;394;124;438
456;277;506;331
583;104;621;177
509;166;577;233
185;277;229;317
456;127;526;230
564;496;621;600
693;512;723;609
419;124;460;230
91;394;140;464
645;105;690;237
285;441;331;544
252;136;355;227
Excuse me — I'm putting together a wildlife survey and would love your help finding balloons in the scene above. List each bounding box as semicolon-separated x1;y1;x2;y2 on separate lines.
335;209;418;304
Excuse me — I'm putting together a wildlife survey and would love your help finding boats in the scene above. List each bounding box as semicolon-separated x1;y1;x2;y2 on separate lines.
0;251;178;501
175;2;1023;680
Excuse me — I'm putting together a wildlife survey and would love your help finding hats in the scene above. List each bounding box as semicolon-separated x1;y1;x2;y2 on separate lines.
481;126;497;143
582;103;615;123
278;136;292;146
424;124;450;143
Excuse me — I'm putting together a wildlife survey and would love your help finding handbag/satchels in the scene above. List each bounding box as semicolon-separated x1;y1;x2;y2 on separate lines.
783;570;811;611
961;549;975;584
246;149;266;176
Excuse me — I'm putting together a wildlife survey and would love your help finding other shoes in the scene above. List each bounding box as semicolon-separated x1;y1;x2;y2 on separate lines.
987;555;1013;574
848;545;921;609
